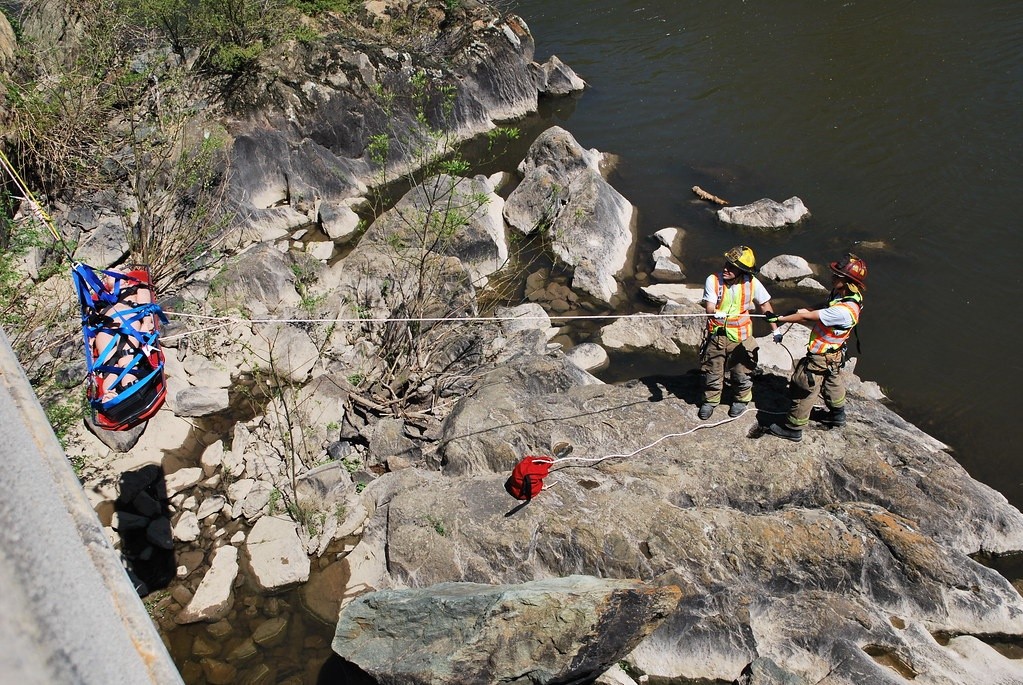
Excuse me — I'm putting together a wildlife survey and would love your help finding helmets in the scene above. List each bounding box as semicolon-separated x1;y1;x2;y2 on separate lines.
725;245;755;271
831;253;868;287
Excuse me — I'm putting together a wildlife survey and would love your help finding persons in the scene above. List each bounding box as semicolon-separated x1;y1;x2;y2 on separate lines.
698;245;783;419
768;252;868;441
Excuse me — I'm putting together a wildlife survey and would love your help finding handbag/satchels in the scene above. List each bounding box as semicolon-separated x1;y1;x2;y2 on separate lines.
508;455;553;499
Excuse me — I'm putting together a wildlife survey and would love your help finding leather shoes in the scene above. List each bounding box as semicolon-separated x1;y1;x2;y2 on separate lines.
728;402;748;416
699;405;713;420
771;422;802;441
818;407;847;425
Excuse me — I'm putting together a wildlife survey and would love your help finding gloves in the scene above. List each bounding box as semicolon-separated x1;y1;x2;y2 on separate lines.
713;310;727;319
771;327;783;344
783;309;798;316
762;312;778;322
